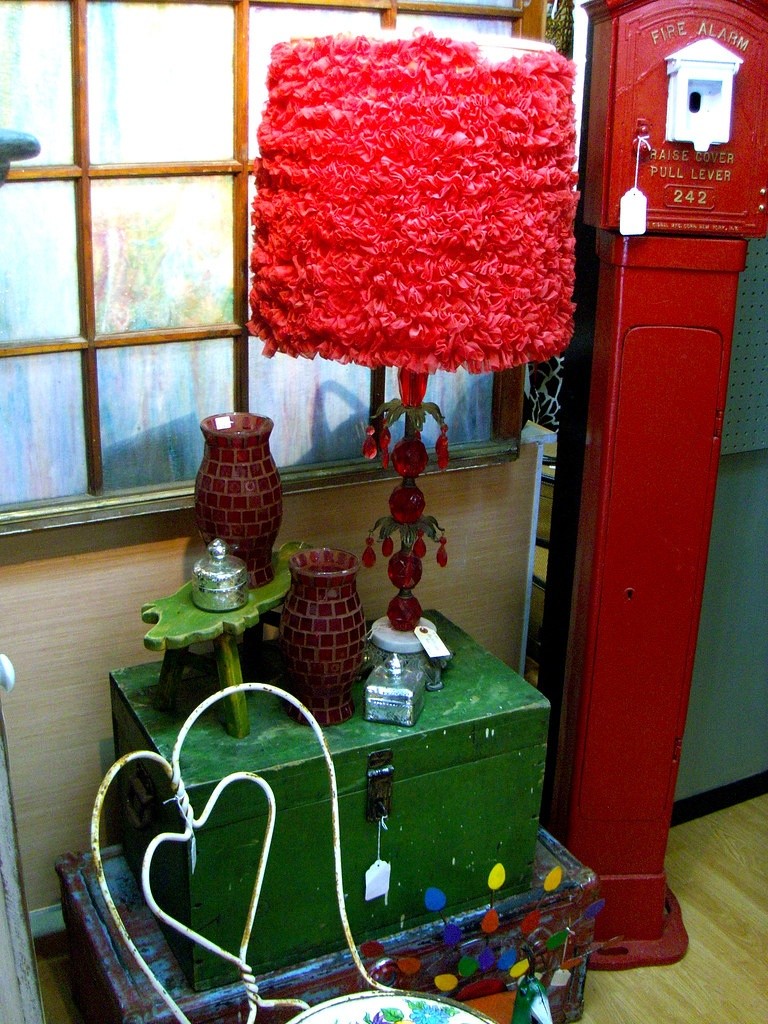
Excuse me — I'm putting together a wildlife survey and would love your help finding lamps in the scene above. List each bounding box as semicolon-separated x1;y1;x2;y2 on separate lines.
246;29;582;654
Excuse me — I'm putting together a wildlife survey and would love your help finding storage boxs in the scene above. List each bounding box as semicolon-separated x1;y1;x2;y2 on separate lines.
54;824;601;1023
109;610;552;993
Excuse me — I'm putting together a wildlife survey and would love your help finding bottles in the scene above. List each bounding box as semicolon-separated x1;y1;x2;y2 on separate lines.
193;411;284;590
279;549;369;727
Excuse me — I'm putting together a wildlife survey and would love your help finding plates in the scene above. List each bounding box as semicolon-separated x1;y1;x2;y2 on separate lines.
284;990;501;1024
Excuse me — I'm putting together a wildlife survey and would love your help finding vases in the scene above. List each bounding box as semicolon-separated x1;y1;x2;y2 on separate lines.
277;547;367;727
194;413;284;588
